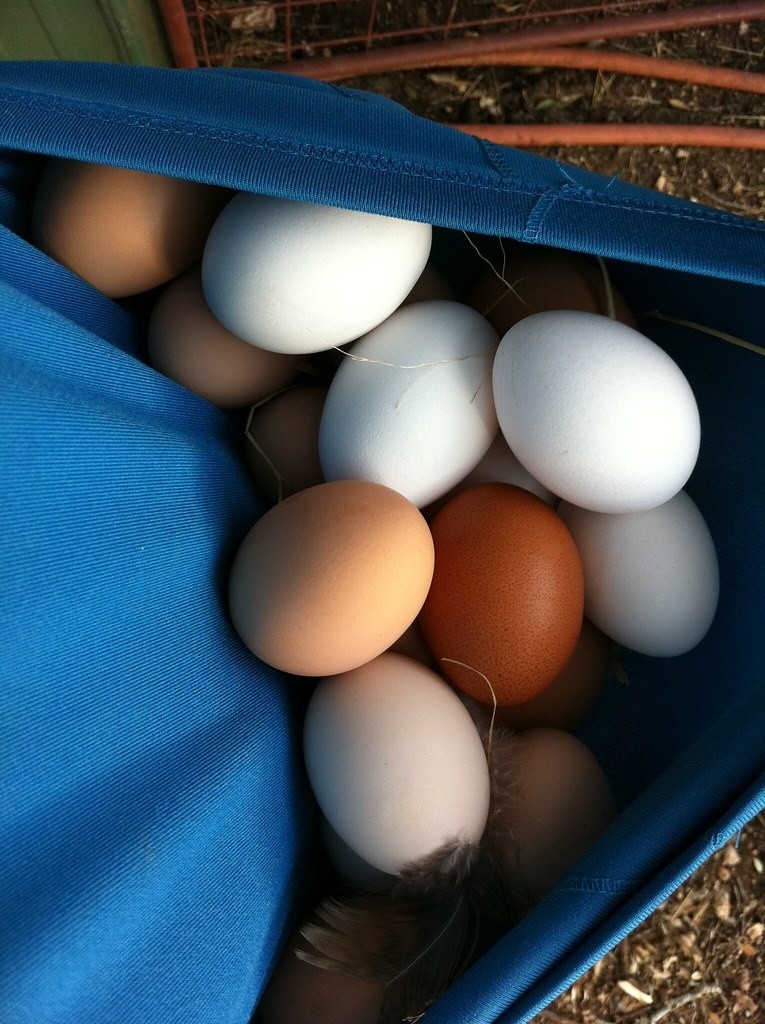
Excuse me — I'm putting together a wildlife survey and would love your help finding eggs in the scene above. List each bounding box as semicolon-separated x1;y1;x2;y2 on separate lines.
31;155;720;1024
